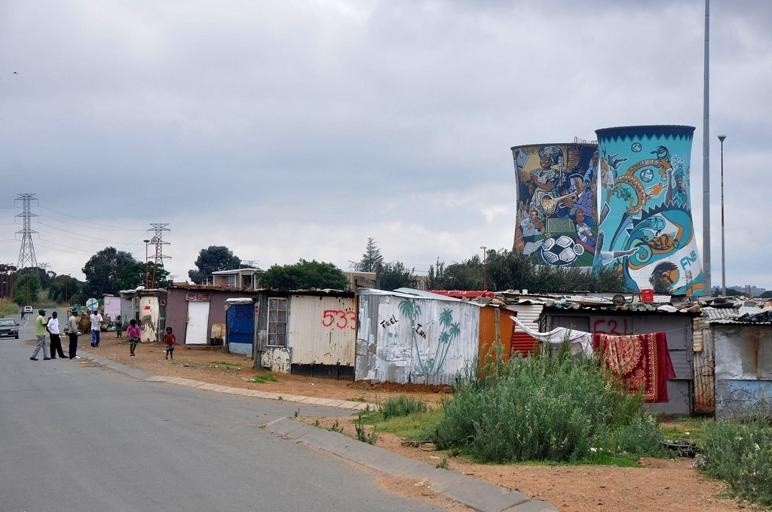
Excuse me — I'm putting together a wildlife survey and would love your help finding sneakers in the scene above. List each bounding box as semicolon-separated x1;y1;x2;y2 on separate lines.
30;355;82;360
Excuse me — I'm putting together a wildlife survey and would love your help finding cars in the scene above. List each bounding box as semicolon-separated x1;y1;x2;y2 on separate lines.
0;319;20;339
23;305;33;314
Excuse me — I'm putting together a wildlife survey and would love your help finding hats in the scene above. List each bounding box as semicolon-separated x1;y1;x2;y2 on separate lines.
38;309;47;312
70;308;79;313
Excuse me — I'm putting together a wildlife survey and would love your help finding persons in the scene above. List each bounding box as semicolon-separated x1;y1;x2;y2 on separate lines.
115;314;122;338
529;148;560;208
573;207;596;245
561;173;594;228
162;326;177;360
126;318;141;357
89;309;105;347
20;309;26;320
137;319;143;344
45;311;68;359
29;309;52;361
520;210;546;258
67;310;82;360
